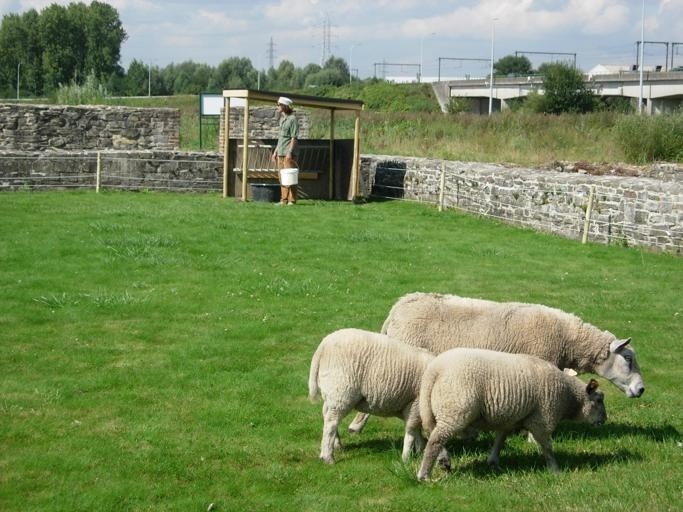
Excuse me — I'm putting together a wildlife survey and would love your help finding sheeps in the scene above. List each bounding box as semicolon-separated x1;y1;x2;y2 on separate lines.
348;292;645;443
307;328;477;465
417;347;607;482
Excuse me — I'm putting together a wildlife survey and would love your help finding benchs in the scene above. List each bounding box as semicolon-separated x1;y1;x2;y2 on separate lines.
231;166;324;181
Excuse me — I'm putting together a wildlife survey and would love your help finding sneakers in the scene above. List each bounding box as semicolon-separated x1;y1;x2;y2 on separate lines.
274;201;294;206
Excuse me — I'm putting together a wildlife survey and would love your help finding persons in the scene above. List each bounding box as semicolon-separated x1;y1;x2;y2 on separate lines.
271;96;299;206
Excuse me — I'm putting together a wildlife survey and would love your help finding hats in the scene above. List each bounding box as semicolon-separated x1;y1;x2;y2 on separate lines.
278;97;292;106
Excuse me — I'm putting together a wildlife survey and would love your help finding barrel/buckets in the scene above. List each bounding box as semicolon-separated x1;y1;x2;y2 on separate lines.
279;159;300;186
